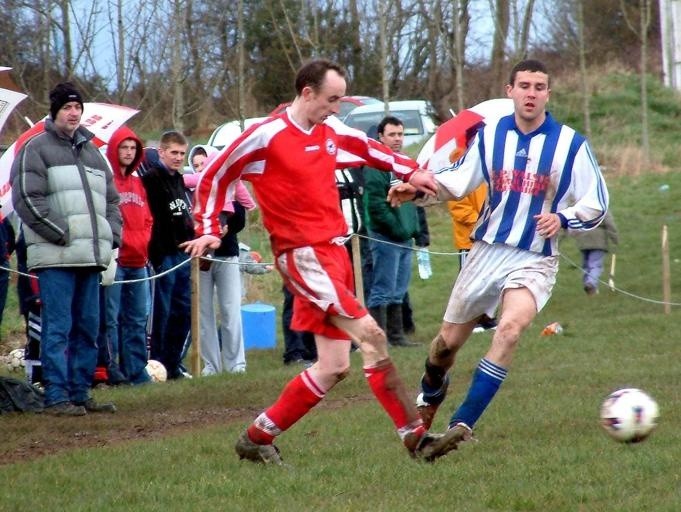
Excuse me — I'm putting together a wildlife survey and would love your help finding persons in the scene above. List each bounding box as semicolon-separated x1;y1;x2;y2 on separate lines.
283;116;430;367
1;146;44;393
179;60;468;465
98;126;153;387
386;61;609;443
577;210;619;295
449;121;497;329
10;84;125;415
182;144;258;376
142;130;193;379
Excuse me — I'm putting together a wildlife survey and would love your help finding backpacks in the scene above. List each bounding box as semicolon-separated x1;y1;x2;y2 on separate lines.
0;375;44;414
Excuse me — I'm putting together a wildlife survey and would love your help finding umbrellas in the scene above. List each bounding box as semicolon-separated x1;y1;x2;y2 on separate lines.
416;98;514;171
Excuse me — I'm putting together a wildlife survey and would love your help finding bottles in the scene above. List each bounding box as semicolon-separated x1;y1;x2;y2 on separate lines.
416;244;433;281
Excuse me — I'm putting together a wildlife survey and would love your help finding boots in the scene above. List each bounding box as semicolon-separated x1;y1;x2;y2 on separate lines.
387;303;422;346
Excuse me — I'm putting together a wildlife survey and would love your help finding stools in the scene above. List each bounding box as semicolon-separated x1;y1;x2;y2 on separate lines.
238;302;277;352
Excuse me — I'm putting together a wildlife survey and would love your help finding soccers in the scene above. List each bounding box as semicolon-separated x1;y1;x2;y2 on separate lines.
5;348;25;373
146;360;168;383
600;387;659;441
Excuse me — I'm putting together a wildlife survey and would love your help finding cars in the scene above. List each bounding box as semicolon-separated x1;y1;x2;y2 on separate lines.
261;95;387;119
344;100;436;147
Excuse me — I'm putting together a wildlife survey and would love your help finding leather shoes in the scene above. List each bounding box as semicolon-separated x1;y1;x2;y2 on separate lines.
73;398;116;413
49;400;87;415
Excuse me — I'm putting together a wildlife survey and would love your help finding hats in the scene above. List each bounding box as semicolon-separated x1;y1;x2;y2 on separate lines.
50;81;84;121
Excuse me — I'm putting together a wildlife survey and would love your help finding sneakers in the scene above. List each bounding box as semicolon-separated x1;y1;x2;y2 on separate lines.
448;421;474;440
403;425;466;464
290;353;314;367
585;282;596;295
33;381;46;393
415;392;441;430
235;427;285;468
